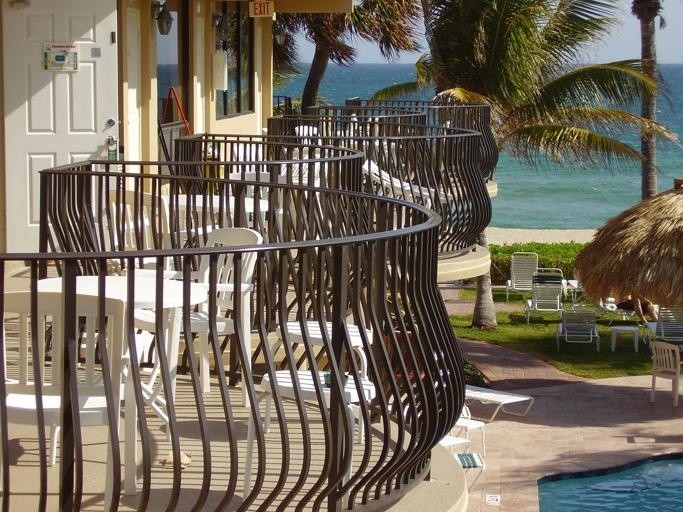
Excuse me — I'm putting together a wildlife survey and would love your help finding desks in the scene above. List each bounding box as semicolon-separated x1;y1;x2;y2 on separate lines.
163;195;270;231
30;274;210;466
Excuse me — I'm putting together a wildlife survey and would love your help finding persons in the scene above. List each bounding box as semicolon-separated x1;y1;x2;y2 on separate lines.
613;285;659;323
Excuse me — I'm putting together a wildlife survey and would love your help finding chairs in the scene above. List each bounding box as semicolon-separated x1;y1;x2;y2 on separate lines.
450;419;486;459
3;291;151;512
505;251;683;408
464;382;535;423
121;227;264;408
439;435;471;453
108;190;170;267
452;452;485;493
227;171;287;208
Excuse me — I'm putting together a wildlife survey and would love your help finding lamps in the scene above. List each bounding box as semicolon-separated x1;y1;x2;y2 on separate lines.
150;1;173;34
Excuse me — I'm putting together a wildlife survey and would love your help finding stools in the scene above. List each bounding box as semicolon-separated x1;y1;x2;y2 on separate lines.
242;370;377;509
263;319;374;446
